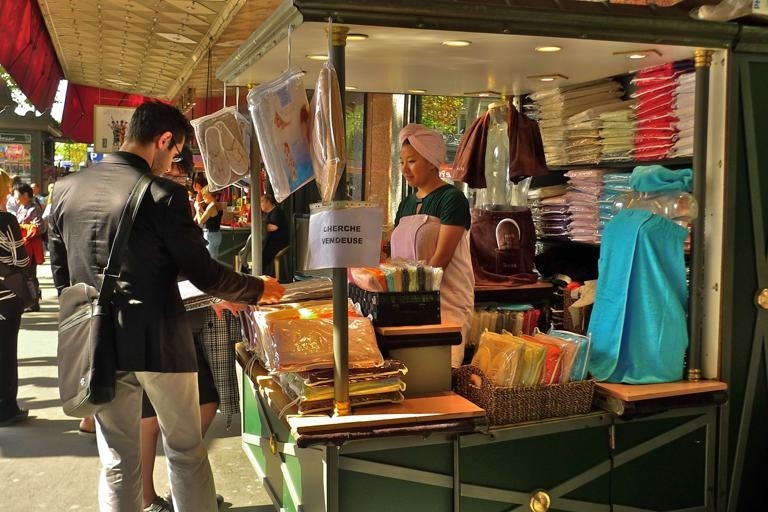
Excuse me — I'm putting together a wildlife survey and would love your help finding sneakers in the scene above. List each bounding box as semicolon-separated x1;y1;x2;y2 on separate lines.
0;408;28;427
142;496;173;512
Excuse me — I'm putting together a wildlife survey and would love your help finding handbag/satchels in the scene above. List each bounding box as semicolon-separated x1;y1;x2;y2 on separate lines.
56;282;115;418
3;272;33;307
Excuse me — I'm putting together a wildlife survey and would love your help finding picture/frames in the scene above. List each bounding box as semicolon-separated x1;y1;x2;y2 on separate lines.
94;105;134;153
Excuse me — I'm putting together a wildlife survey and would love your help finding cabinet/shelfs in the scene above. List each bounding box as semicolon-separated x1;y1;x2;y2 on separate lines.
512;59;692;286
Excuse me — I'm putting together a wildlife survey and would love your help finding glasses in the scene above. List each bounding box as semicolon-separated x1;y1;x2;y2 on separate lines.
161;173;192;184
172;137;184;162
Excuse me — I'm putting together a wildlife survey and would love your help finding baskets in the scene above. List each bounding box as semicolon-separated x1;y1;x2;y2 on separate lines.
450;364;596;427
349;284;441;328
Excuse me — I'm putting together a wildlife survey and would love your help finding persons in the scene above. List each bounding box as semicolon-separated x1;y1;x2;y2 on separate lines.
48;103;284;512
386;123;475;369
260;191;290;280
193;184;223;264
453;100;550;283
191;173;210;229
139;145;250;509
0;168;30;425
4;174;57;315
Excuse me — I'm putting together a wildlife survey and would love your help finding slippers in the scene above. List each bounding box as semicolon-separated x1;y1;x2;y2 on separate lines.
215;121;249;176
204;127;231;186
